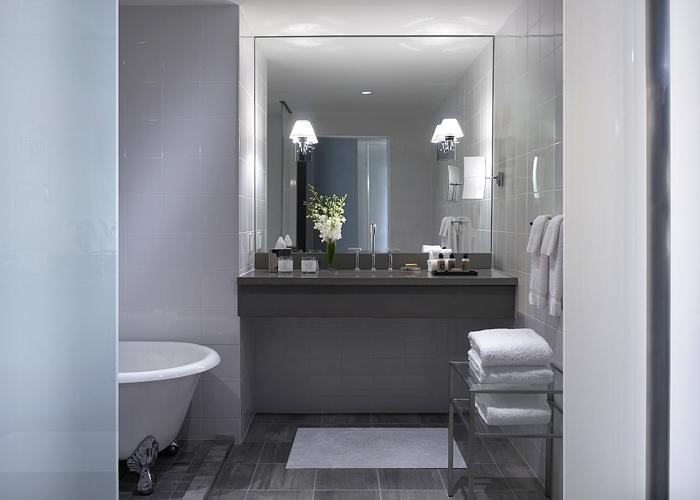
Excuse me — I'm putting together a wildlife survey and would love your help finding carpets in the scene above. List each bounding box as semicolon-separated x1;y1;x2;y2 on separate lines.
284;426;468;470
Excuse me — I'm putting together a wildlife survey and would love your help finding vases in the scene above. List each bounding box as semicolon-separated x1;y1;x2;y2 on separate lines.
327;241;336;269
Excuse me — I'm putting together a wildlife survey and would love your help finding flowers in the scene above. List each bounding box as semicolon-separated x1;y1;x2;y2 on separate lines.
303;184;347;243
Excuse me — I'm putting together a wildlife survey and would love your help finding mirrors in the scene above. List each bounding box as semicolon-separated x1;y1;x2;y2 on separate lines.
253;35;496;269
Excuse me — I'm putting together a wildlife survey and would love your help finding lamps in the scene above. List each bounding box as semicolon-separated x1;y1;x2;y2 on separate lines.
439;119;464;150
431;124;448;153
446;164;462;202
291;136;318;154
288;119;313;153
462;156;503;200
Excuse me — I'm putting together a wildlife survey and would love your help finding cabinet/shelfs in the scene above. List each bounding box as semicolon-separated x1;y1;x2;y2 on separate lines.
446;359;562;499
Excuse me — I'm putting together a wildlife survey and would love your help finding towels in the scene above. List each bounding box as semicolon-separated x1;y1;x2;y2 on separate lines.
439;217;449;248
458;217;471;254
526;214;551;310
540;214;563;317
442;217;458;253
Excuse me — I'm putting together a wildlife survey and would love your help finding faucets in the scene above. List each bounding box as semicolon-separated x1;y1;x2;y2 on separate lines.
368;222;378;272
